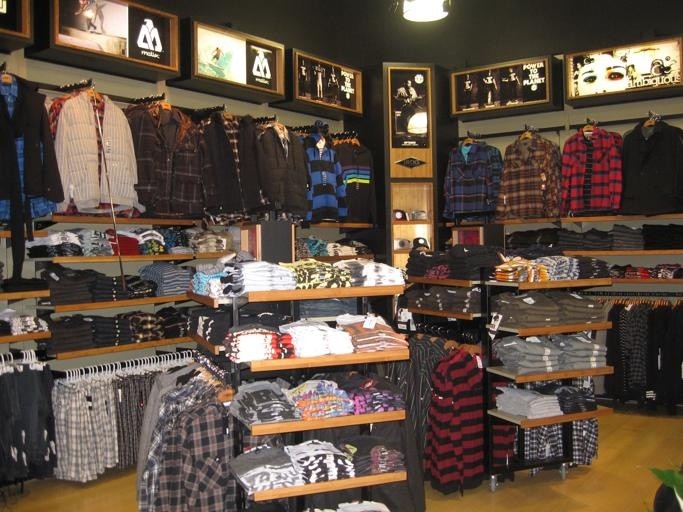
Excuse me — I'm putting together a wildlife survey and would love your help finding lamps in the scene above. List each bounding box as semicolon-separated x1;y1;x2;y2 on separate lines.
81;0;96;18
407;108;427;134
402;0;449;22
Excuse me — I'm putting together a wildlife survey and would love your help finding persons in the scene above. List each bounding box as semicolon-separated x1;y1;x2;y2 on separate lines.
462;73;475;108
481;68;499;108
326;66;341;105
572;49;631;98
297;58;309;96
500;66;521;106
207;45;224;66
394;78;425;127
312;62;327;98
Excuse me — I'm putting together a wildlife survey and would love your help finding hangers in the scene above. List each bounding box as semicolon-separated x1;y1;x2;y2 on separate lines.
577;118;605;138
458;129;483;150
639;111;664;132
517;124;547;147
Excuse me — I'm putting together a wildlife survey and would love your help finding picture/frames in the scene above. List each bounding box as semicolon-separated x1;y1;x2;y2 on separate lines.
381;61;437;182
